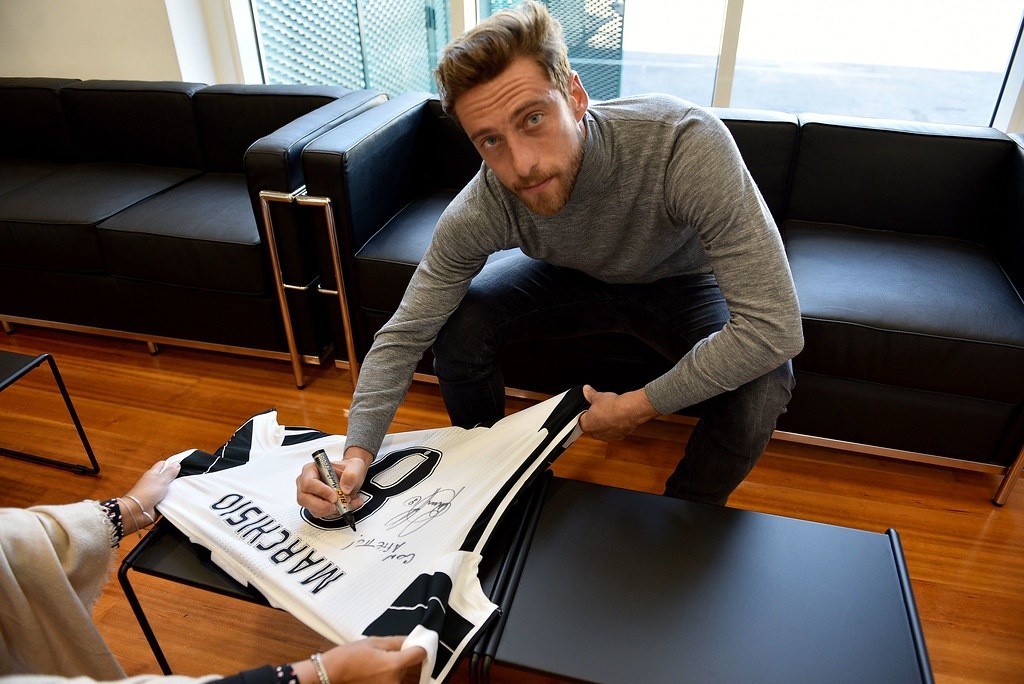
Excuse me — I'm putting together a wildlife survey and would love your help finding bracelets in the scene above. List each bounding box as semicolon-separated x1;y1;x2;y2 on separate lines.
112;497;142;542
311;652;330;684
125;495;157;532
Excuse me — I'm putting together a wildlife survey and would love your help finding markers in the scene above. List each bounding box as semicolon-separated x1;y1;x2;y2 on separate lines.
312;448;357;532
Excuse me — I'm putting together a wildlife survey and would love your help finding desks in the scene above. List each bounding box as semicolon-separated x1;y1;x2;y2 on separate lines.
483;470;936;684
117;409;550;684
0;351;101;476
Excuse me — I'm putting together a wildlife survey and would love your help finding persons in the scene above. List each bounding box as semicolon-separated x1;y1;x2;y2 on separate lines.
296;0;805;520
0;460;428;684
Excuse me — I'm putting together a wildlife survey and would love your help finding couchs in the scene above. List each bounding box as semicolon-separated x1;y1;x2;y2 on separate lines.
0;74;390;390
299;89;1024;506
